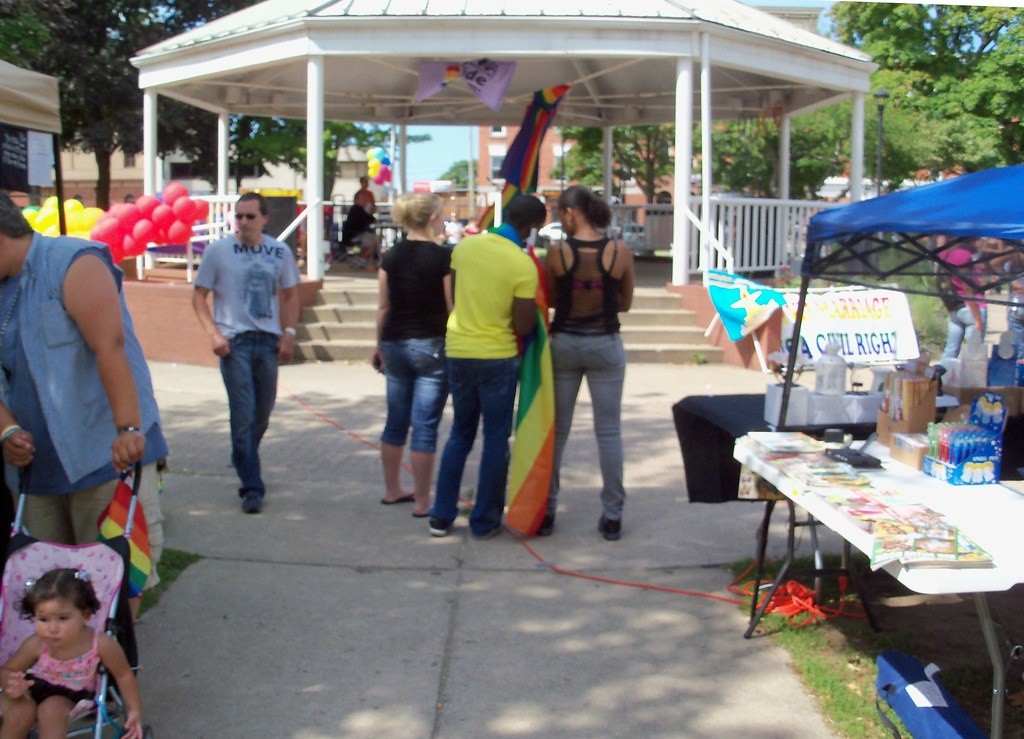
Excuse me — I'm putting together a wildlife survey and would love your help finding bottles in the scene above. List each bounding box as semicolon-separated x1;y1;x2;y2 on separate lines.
815;344;846;393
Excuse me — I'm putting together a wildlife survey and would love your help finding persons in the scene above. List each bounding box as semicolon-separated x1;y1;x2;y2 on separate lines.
937;231;987;357
342;193;377;272
354;176;376;215
990;238;1024;358
125;194;135;203
535;185;634;541
373;194;453;517
0;193;169;719
426;195;547;535
0;568;143;738
192;191;302;513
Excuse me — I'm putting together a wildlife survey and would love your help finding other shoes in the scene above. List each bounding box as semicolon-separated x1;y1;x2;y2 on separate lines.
538;512;556;535
598;515;621;539
428;516;449;536
241;493;262;512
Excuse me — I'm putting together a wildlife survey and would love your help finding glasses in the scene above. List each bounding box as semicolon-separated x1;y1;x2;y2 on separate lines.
234;214;260;220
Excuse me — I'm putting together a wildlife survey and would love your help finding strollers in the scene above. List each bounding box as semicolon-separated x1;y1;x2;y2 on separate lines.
0;451;155;739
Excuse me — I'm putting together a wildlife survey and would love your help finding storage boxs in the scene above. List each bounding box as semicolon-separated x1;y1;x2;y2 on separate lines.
890;432;929;471
764;340;1024;486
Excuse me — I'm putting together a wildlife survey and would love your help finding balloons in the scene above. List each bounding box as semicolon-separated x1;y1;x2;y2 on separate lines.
91;180;209;264
366;147;392;185
21;198;106;241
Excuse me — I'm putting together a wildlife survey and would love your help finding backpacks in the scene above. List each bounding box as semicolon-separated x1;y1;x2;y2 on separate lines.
935;263;965;310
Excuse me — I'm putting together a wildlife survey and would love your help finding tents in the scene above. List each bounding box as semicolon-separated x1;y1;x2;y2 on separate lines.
745;161;1024;634
0;58;68;236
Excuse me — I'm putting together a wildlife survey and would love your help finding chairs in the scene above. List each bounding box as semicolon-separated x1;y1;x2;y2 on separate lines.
327;221;372;268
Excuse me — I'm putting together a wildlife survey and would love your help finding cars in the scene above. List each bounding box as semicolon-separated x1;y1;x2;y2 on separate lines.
537;222;570;242
618;223;647;250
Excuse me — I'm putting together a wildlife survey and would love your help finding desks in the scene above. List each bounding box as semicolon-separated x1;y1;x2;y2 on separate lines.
368;222;403;247
672;394;877;504
944;385;1023;417
733;431;1023;739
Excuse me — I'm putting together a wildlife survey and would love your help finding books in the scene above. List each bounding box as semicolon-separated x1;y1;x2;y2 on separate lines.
746;432;994;572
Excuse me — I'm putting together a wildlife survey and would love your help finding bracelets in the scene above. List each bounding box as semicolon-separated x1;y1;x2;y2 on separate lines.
0;425;22;442
285;326;296;335
117;424;141;434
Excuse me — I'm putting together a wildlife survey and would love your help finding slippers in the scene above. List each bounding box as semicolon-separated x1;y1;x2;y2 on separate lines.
412;511;429;517
381;492;415;504
